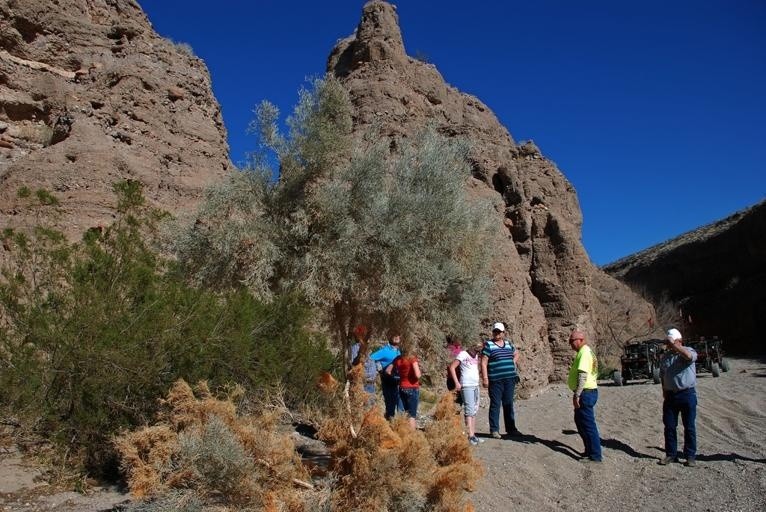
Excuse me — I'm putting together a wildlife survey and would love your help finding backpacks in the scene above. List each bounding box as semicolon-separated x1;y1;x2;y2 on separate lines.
447;365;460;390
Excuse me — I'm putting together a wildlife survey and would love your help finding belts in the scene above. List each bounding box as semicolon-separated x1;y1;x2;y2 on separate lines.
367;381;374;384
573;389;597;392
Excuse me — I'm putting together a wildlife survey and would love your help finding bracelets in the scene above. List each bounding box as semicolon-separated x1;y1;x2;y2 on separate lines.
483;376;489;379
669;342;677;346
572;392;581;399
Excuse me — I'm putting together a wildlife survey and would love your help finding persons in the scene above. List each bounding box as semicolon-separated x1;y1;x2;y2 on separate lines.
480;321;524;439
391;349;422;420
349;325;379;411
446;341;464;415
658;326;699;469
449;339;485;445
565;331;604;463
371;331;402;422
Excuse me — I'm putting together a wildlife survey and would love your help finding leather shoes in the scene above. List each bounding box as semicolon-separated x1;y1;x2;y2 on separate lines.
579;458;602;463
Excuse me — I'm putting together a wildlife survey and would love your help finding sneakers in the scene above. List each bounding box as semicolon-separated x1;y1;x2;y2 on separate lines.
469;437;478;445
489;431;502;439
659;456;678;465
470;435;485;443
686;458;696;467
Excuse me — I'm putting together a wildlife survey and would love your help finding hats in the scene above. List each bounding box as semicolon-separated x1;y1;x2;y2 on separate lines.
492;322;505;332
667;328;683;341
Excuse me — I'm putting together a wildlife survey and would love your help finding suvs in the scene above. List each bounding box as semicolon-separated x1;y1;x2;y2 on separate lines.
614;341;666;386
702;341;729;372
679;340;721;379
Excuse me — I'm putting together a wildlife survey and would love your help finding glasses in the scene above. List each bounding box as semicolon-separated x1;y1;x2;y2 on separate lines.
569;339;581;344
